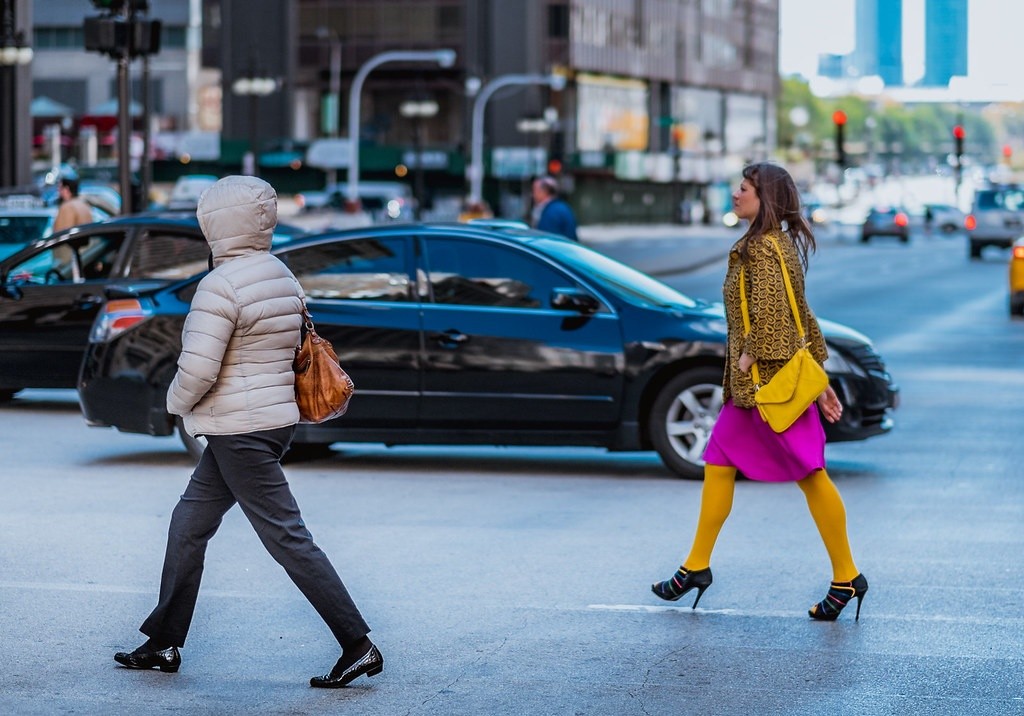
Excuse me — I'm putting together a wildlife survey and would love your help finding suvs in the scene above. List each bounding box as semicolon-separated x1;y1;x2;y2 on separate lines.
965;186;1024;260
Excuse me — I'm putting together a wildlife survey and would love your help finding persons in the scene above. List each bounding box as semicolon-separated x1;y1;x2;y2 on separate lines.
532;176;578;241
651;164;868;624
114;175;384;687
52;177;94;265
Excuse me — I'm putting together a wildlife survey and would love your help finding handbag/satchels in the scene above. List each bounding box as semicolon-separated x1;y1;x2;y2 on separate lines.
755;341;829;436
294;328;354;424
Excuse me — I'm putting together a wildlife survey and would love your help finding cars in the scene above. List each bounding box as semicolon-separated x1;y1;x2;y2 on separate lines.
0;182;125;297
861;205;911;245
920;204;968;235
79;220;901;480
326;184;415;225
1;178;317;406
1010;235;1024;318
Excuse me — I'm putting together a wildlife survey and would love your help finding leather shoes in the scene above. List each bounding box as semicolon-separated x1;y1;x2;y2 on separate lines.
310;644;383;687
113;645;181;672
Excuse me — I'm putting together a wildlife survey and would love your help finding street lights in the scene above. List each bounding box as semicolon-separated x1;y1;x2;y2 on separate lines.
344;49;459;202
471;70;569;204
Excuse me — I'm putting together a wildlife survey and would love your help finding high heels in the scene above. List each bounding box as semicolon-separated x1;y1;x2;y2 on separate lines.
652;565;713;608
810;573;868;621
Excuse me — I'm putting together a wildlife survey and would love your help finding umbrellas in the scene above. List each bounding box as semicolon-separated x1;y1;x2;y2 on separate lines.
31;95;144;117
